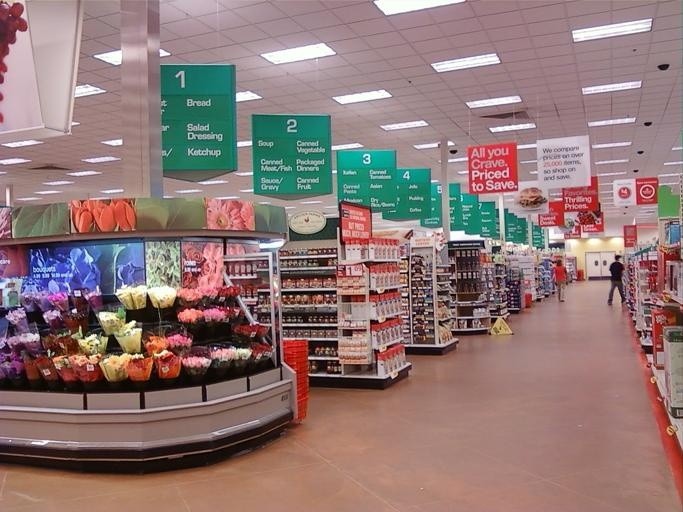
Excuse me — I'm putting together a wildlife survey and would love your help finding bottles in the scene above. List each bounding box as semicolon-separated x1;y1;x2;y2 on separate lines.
253;245;343;376
456;261;481;293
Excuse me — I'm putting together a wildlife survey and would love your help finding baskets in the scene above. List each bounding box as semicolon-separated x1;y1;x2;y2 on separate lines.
282;338;309;420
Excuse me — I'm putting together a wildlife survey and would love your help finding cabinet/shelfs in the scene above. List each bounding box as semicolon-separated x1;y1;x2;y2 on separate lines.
622;216;683;453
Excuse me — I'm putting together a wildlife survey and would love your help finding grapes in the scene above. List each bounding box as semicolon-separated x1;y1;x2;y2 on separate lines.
0;0;28;123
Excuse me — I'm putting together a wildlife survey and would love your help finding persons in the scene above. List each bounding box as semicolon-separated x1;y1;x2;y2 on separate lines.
606;255;627;305
549;259;568;302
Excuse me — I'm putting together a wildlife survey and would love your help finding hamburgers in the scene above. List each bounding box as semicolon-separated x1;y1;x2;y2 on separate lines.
519;188;547;208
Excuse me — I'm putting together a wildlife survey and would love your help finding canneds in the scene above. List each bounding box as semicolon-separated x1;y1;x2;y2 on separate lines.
327;361;341;374
312;361;316;373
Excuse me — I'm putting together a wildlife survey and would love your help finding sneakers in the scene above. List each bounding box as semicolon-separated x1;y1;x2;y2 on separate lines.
621;298;626;303
607;301;612;305
